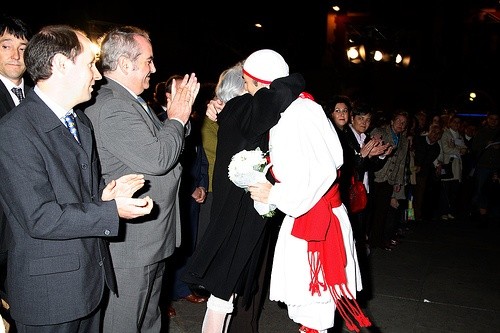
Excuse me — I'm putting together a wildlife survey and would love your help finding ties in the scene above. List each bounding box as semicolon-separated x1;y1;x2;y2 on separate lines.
11;87;25;103
136;95;153;120
64;113;82;145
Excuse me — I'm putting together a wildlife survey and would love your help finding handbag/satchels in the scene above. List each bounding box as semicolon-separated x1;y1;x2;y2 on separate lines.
337;168;367;212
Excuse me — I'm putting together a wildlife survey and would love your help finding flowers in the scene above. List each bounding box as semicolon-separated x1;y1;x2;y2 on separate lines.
228;148;278;218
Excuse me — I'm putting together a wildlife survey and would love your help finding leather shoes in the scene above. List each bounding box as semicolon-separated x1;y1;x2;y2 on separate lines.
168;307;176;317
185;295;208;303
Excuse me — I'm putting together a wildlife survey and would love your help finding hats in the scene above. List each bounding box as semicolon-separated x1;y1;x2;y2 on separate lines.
241;49;290;84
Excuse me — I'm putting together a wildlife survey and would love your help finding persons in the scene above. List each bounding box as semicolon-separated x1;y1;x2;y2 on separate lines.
206;48;371;333
85;26;201;333
327;98;389;224
370;111;409;251
407;110;500;224
182;58;314;333
349;104;394;233
197;118;219;248
0;19;34;118
0;25;154;333
163;75;205;318
